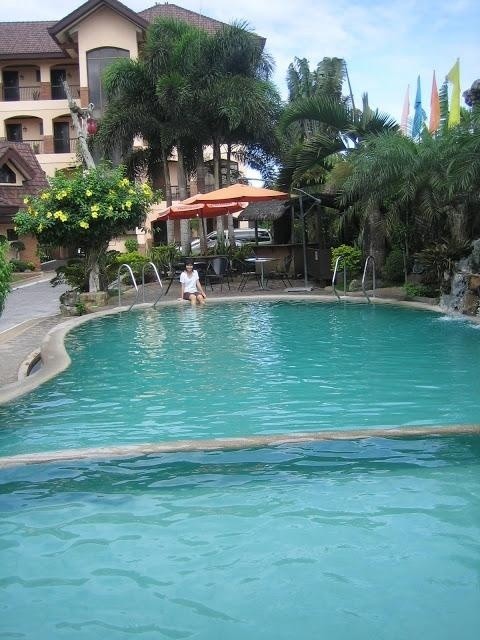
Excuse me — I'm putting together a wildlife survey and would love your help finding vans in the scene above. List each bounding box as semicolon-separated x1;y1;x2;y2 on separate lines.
175;229;273;253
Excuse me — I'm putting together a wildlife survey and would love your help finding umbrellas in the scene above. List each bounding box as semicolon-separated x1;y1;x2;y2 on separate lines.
192;182;287;205
156;189;250;256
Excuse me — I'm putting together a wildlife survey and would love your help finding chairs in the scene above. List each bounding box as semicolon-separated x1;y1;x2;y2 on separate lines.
164;253;294;296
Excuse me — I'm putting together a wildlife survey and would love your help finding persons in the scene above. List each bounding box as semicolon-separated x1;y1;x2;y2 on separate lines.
178;258;209;309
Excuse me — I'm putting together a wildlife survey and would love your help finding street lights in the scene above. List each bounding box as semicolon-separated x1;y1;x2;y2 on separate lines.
238;177;321;290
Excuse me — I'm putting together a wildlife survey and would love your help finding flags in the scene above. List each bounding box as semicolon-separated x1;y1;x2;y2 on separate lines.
428;66;442;137
445;57;464;131
399;83;411;137
411;73;427;137
